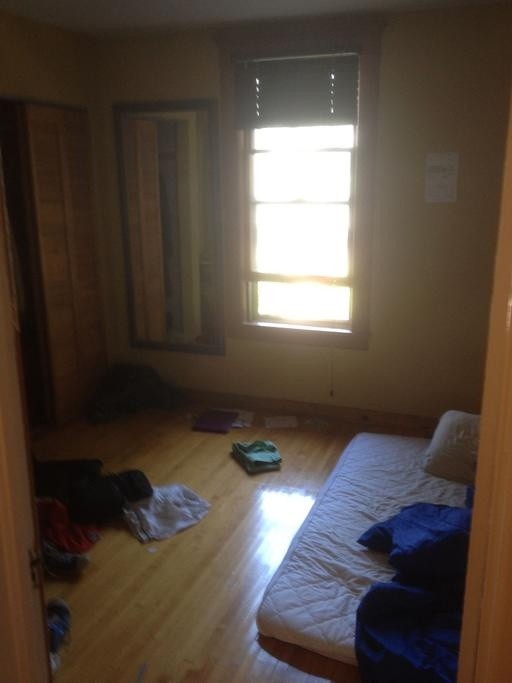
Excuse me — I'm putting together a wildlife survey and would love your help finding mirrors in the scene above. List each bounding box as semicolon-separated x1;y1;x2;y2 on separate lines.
115;99;230;357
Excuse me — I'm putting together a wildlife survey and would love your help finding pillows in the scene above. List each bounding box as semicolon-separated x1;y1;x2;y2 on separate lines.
423;407;482;487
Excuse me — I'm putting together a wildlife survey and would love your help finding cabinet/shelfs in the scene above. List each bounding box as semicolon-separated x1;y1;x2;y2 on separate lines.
3;96;111;432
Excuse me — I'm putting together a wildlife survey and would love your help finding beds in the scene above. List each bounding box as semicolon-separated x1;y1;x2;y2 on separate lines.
253;432;469;670
